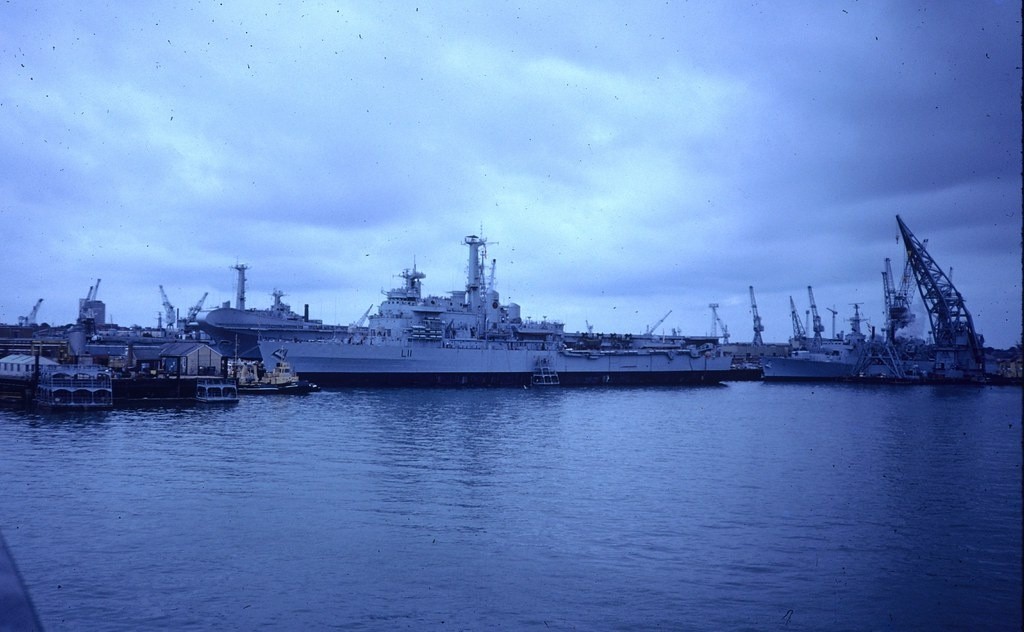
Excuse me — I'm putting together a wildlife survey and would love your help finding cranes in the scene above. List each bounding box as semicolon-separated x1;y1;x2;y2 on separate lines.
708;213;984;382
185;290;208;327
77;278;101;329
19;297;44;326
158;284;175;332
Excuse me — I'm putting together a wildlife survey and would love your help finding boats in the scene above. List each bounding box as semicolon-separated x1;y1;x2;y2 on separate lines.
261;346;302;385
192;376;242;407
34;364;113;411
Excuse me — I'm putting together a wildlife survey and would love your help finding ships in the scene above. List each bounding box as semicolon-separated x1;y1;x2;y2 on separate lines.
256;231;736;383
